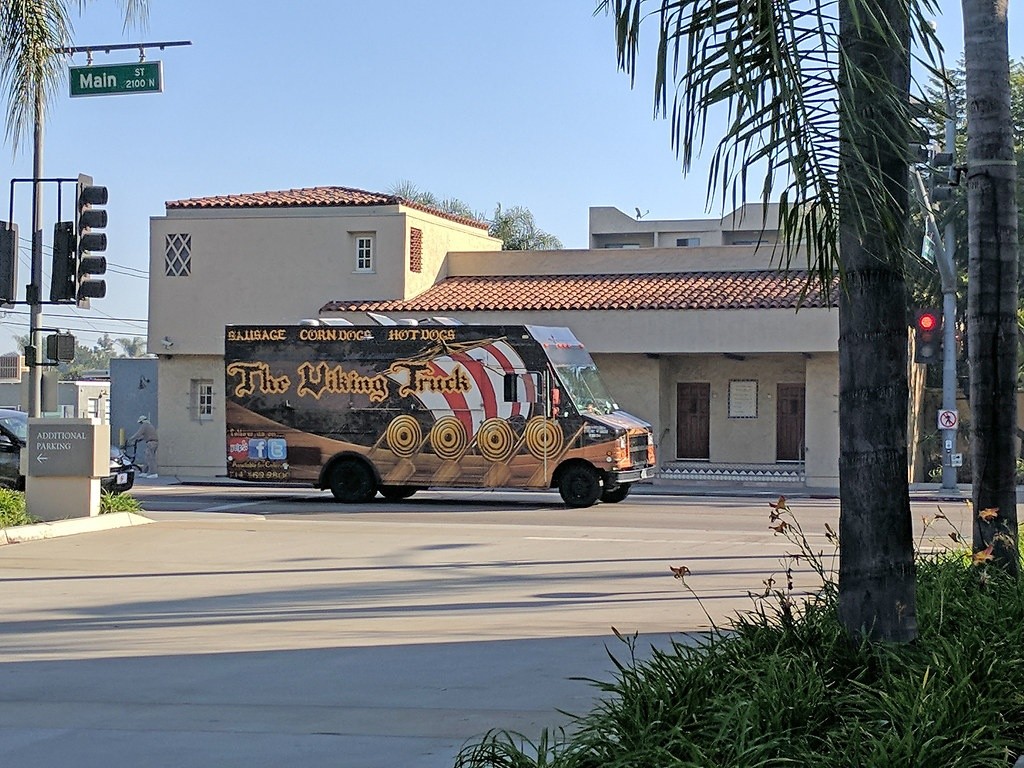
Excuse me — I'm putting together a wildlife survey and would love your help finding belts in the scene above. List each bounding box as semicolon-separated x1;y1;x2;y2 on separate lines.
146;440;158;443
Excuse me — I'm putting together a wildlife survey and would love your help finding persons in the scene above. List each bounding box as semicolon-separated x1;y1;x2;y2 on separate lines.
127;415;159;479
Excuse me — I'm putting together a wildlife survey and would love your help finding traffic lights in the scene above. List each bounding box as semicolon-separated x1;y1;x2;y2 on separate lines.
914;309;942;363
929;150;954;203
76;173;108;309
908;101;930;164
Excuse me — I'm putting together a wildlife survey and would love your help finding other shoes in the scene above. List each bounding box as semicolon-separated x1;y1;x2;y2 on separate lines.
147;474;158;478
139;472;147;477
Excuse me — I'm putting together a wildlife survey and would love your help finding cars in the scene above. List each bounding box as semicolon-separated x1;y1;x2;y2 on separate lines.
0;409;135;493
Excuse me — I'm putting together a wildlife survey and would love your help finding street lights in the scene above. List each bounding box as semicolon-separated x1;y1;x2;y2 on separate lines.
917;17;961;488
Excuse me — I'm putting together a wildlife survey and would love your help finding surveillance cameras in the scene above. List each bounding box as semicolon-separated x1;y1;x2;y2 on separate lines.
160;340;174;346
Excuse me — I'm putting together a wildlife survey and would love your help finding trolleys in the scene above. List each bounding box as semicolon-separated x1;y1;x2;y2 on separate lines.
119;439;142;474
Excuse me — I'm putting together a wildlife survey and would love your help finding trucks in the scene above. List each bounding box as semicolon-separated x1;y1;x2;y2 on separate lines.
224;311;656;508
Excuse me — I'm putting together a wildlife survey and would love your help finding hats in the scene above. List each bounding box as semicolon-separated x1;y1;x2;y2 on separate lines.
137;415;147;423
